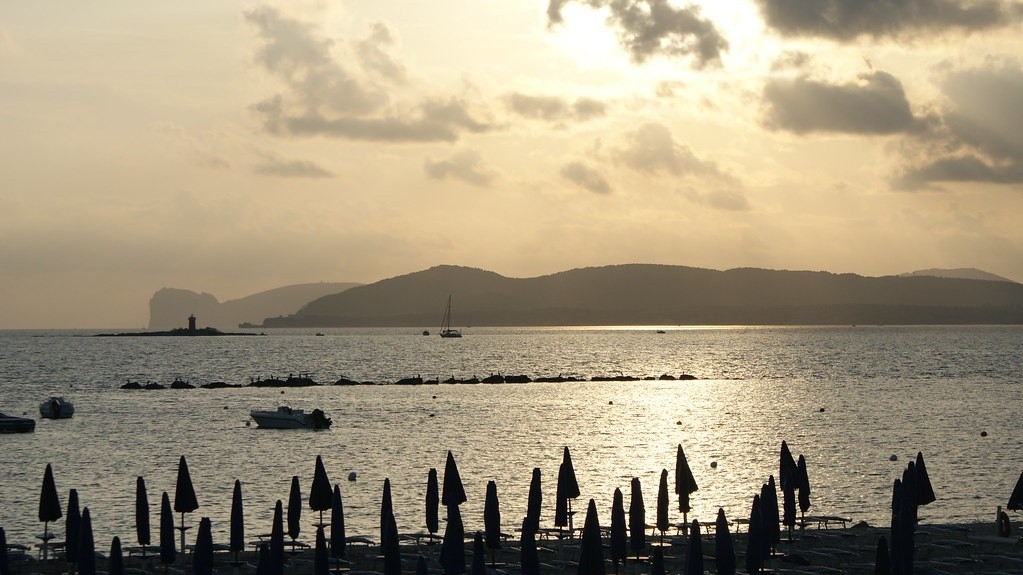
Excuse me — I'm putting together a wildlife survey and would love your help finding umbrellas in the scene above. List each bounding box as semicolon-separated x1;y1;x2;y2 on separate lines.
38;441;1023;575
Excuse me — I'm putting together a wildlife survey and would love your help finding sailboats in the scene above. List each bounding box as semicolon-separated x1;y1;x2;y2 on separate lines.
438;293;462;338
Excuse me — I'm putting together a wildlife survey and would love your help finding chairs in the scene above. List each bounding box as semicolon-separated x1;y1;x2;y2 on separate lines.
6;515;1023;575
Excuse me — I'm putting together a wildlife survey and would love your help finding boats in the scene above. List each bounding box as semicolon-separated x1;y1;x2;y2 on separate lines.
316;332;325;336
39;396;75;419
0;413;36;433
259;332;267;336
248;406;332;430
